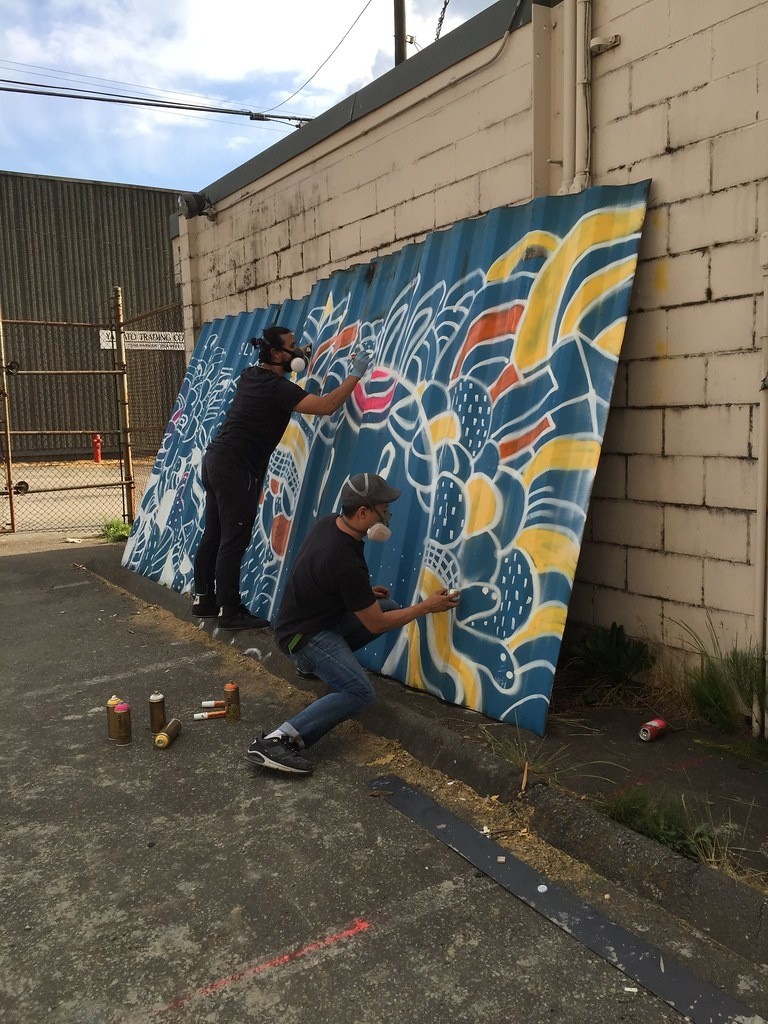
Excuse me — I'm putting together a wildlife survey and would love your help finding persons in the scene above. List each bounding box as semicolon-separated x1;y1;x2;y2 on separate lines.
187;325;373;629
245;472;462;774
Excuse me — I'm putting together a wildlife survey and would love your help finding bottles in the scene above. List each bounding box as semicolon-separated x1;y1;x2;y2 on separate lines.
194;711;225;720
106;695;123;741
149;690;166;735
442;588;460;602
155;718;182;748
224;680;241;726
202;701;225;708
115;701;132;747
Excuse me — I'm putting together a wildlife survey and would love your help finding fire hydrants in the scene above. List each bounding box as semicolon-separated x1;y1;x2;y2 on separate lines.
92;433;102;463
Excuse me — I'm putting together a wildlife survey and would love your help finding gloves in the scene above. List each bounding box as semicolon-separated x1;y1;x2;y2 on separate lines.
349;350;372;379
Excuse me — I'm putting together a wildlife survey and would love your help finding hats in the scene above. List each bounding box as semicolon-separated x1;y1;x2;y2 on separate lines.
341;473;401;507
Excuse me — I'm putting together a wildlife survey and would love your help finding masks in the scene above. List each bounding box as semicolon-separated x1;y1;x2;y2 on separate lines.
284;350;309;373
366;522;392;542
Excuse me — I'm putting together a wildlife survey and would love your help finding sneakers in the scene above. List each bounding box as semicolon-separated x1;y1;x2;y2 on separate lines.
192;594;218;618
243;732;315;773
217;604;271;630
295;667;323;682
